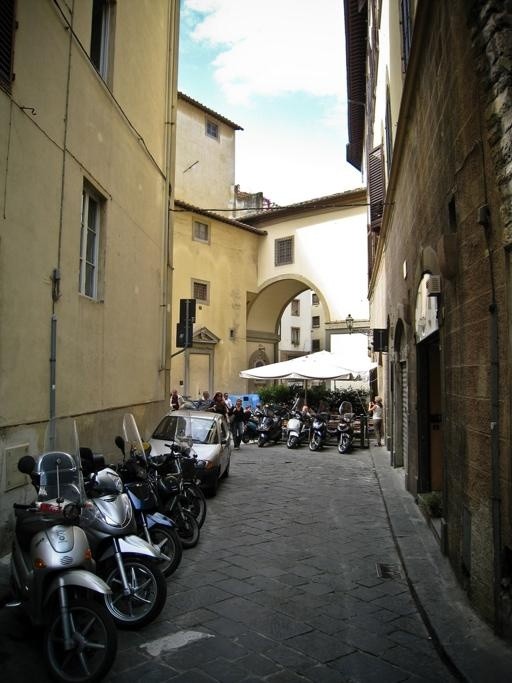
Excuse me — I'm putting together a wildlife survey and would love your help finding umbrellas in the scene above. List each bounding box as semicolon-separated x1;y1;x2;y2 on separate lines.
239;350;378;406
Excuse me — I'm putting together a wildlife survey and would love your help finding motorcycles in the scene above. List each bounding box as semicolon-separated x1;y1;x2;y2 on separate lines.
241;402;355;454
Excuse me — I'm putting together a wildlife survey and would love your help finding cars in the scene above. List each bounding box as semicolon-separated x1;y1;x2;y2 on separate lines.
147;410;231;498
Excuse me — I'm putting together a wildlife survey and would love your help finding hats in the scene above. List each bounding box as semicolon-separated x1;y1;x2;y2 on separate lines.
375;396;382;402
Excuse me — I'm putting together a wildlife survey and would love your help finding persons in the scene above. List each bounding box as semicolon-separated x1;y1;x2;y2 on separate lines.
198;391;263;450
291;392;302;411
369;396;383;446
170;389;179;410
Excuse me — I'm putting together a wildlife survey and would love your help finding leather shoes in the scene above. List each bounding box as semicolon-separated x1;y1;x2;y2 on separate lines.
374;443;381;446
